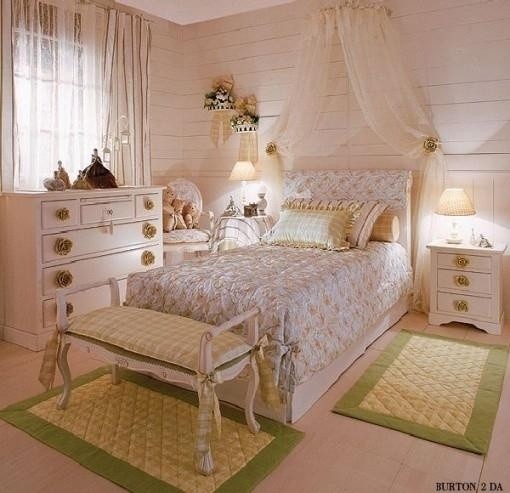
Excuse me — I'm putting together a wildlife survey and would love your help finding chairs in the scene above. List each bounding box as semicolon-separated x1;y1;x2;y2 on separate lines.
162;177;214;258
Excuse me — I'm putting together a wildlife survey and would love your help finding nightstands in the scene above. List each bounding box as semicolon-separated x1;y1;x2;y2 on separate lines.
218;215;270;251
425;242;508;335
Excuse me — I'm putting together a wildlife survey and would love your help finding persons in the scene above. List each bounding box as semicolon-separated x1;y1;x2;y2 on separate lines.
42;147;117;190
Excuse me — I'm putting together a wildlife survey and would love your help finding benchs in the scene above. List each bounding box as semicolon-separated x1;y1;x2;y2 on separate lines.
55;277;261;476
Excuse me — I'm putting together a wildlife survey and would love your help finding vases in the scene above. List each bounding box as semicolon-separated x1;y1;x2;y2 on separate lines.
207;102;233;111
236;124;256;132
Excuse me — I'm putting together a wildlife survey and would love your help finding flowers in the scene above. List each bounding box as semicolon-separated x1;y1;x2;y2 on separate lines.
231;112;259;126
205;83;234;106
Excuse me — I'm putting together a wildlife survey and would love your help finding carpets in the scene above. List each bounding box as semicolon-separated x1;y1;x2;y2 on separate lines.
332;329;510;455
1;363;305;492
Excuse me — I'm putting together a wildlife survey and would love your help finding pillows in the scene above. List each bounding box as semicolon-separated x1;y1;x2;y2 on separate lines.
262;196;389;248
370;214;400;241
264;203;360;251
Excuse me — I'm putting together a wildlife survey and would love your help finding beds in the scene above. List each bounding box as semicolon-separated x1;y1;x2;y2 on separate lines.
126;169;412;425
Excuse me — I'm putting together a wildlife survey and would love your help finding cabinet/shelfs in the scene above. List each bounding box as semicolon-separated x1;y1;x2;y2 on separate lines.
1;184;169;352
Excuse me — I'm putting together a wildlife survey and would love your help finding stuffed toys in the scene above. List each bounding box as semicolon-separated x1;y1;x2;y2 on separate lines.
162;188;200;231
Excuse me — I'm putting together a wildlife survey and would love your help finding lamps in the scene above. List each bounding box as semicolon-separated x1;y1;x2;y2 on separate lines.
435;188;477;244
227;160;259;215
255;181;270;214
